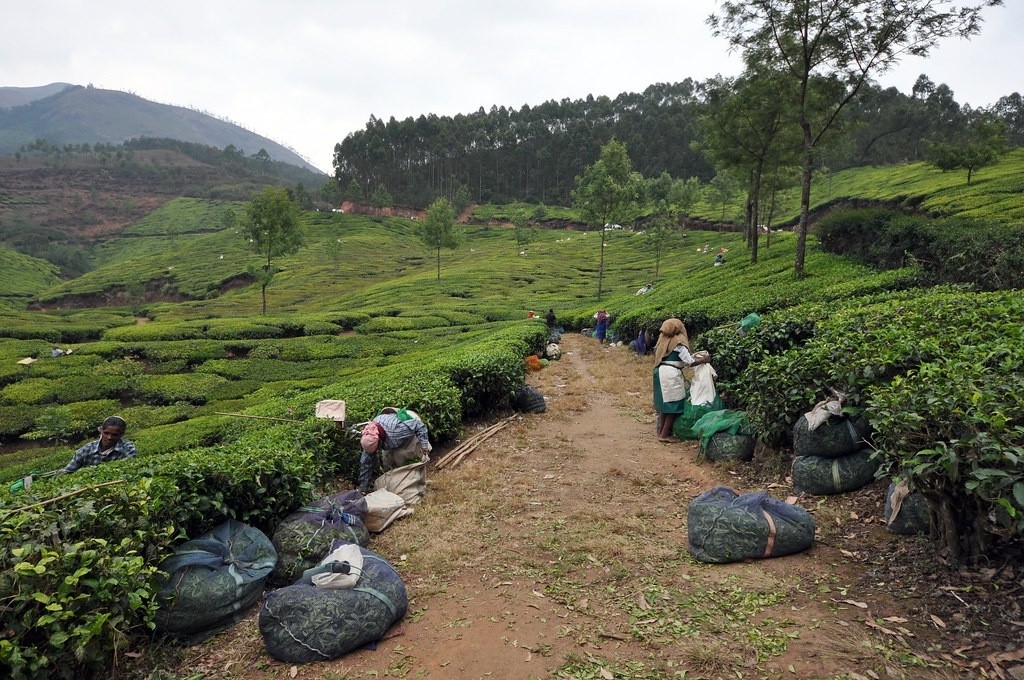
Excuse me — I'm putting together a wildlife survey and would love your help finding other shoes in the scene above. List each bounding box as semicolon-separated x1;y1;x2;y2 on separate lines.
659;435;680;443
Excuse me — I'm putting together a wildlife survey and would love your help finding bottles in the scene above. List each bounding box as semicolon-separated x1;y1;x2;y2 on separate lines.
301;560;351;583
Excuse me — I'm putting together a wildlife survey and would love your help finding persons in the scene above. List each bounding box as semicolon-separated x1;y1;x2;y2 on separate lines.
51;346;63;357
716;255;724;263
594;309;610;344
59;416;139;473
652;318;711;443
358;413;432;495
546;308;556;329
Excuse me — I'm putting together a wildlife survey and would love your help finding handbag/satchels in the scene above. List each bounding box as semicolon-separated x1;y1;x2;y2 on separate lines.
7;468;65;495
714;262;723;267
691;407;755;464
